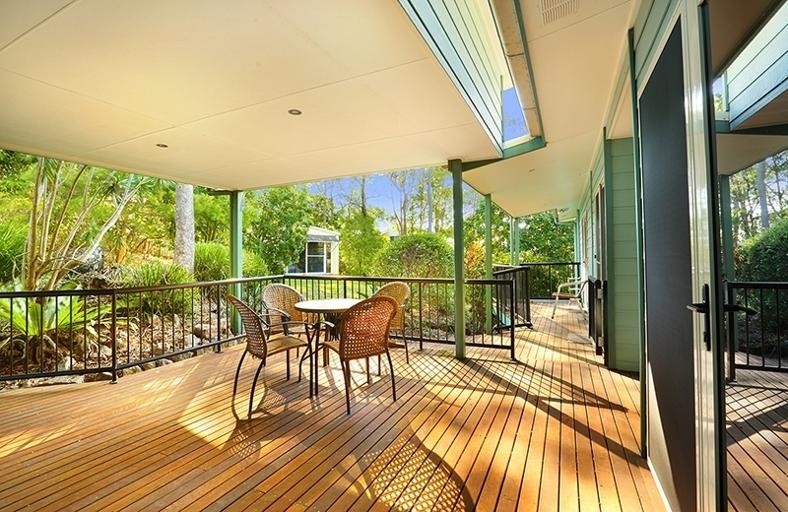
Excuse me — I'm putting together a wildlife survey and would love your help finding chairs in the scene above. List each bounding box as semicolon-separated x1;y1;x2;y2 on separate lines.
551;279;589;319
226;281;412;414
